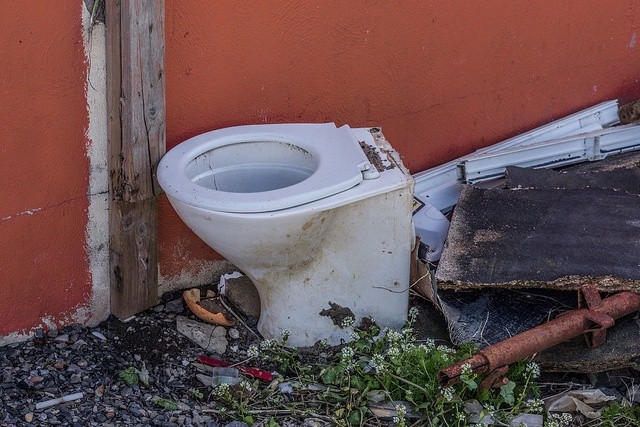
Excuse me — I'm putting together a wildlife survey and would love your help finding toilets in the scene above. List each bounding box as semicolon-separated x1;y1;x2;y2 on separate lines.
155;120;418;352
